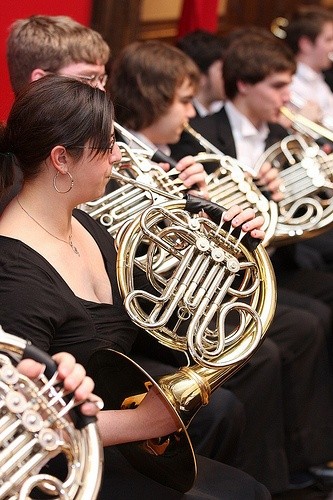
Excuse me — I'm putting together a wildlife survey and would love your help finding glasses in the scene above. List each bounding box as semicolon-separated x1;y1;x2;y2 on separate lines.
62;133;117;154
45;70;108;89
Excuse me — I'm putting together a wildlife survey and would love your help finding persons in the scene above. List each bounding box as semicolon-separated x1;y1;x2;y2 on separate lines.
7;13;288;475
277;6;332;139
111;41;332;476
0;79;273;500
16;347;105;473
178;29;228;123
169;47;332;302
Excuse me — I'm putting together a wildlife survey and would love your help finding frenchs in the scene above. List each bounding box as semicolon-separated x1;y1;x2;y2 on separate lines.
279;104;333;142
90;167;277;492
85;120;278;299
182;121;333;255
0;324;104;500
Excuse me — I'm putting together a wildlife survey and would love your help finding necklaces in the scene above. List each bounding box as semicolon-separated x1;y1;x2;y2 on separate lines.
16;197;82;256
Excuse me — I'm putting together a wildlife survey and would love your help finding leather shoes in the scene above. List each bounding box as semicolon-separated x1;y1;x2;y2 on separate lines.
285;474;316;492
304;465;333;486
